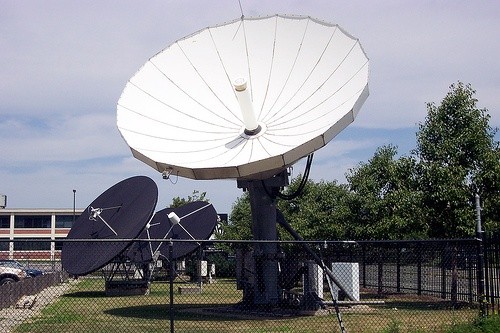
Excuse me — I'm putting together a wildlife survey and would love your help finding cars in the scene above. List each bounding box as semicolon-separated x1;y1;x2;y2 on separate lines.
0;261;27;286
0;259;42;278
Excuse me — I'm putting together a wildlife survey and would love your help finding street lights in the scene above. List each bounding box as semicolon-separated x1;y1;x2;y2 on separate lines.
73;190;77;225
